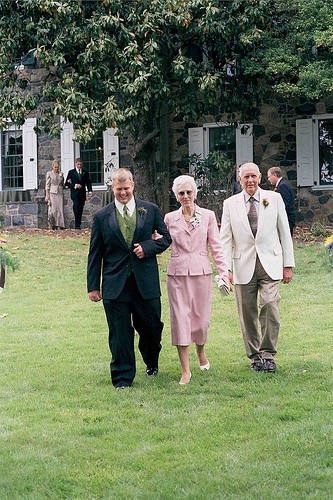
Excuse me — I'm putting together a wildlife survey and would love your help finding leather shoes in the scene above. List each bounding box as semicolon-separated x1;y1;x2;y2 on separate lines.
147;365;157;376
251;362;262;373
115;386;126;390
263;359;276;372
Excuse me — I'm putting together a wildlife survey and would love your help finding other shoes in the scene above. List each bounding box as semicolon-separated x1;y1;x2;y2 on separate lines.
199;363;210;371
179;373;192;385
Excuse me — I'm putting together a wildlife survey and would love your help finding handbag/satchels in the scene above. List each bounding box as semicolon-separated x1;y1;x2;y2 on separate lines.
214;275;229;297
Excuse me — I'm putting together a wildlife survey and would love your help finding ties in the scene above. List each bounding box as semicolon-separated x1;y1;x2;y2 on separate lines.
123;204;130;221
78;169;81;175
247;196;258;236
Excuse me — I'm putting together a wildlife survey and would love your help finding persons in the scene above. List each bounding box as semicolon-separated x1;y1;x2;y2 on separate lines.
221;161;296;374
266;167;296;241
85;169;173;390
150;174;230;385
229;161;253;195
64;158;94;229
44;159;72;231
